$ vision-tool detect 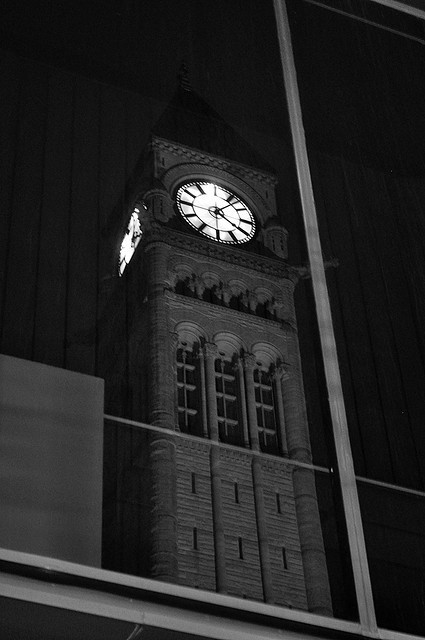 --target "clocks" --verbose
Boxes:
[162,164,272,246]
